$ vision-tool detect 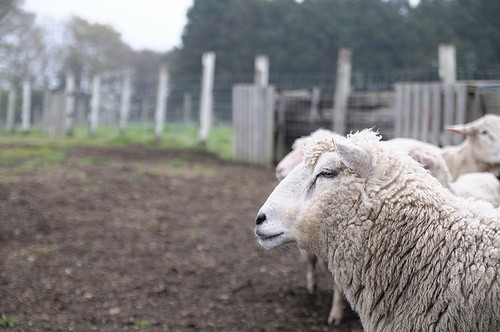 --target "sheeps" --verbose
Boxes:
[254,113,500,332]
